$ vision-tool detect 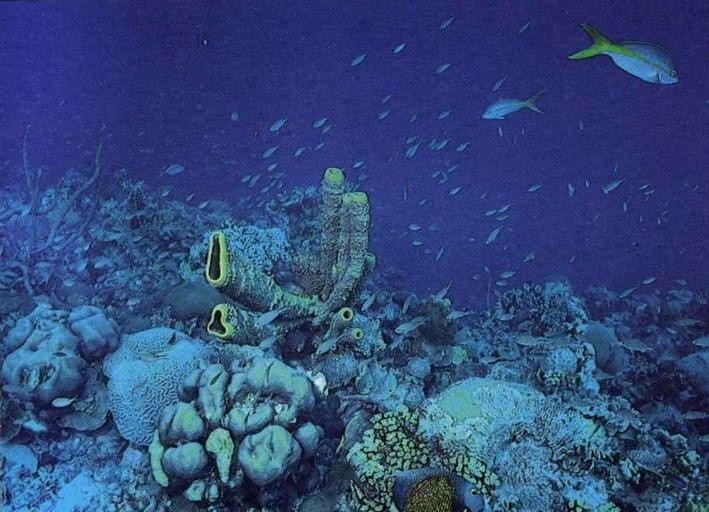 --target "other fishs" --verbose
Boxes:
[0,17,709,512]
